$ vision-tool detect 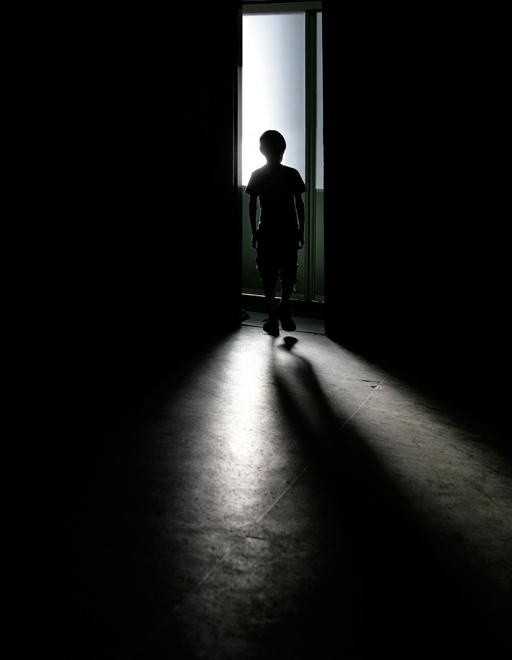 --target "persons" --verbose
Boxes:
[246,130,306,338]
